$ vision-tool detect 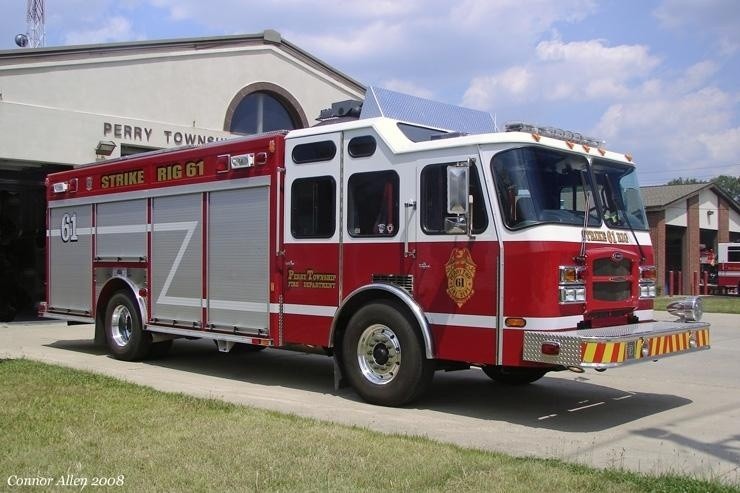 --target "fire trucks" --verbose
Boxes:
[34,85,712,408]
[700,261,740,296]
